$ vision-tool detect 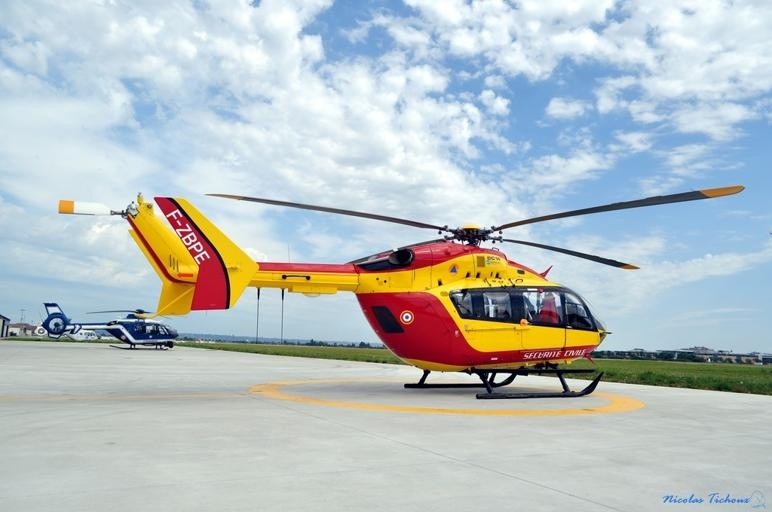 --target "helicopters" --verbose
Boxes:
[58,185,746,399]
[41,303,179,351]
[34,319,101,343]
